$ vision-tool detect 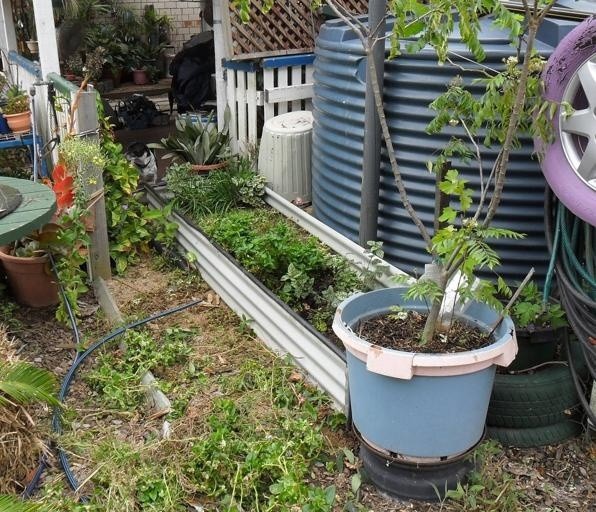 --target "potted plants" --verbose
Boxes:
[235,0,596,472]
[1,83,32,134]
[0,1,176,93]
[146,102,234,179]
[0,205,105,331]
